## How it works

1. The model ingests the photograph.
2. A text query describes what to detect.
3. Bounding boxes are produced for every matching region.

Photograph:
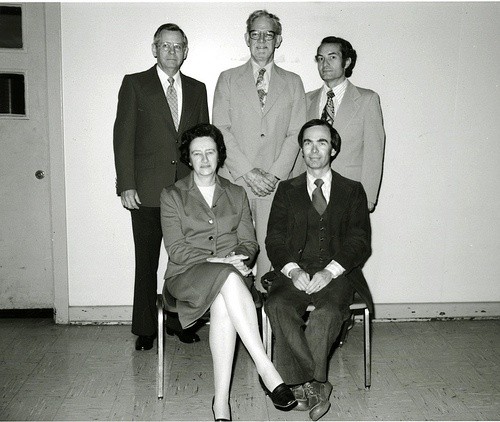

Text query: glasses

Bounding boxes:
[158,43,183,51]
[248,30,278,40]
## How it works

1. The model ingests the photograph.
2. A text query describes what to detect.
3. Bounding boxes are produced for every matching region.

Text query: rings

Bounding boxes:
[318,285,320,288]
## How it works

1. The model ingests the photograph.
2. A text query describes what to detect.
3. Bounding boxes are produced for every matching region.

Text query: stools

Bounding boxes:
[268,293,372,389]
[156,281,269,399]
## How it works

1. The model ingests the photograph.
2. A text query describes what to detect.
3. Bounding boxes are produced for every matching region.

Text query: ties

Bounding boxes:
[256,68,268,108]
[167,77,179,133]
[312,179,327,216]
[321,90,335,127]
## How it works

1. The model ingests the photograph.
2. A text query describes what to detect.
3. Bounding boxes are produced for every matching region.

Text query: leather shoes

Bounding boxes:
[165,318,200,343]
[212,396,232,422]
[259,375,298,412]
[135,334,158,350]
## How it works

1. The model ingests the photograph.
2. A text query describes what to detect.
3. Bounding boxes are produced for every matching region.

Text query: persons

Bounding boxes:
[304,36,385,331]
[263,118,376,421]
[213,10,307,295]
[160,123,299,422]
[113,23,210,350]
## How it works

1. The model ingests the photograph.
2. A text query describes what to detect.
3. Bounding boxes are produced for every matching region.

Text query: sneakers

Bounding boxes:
[302,380,333,421]
[289,384,309,411]
